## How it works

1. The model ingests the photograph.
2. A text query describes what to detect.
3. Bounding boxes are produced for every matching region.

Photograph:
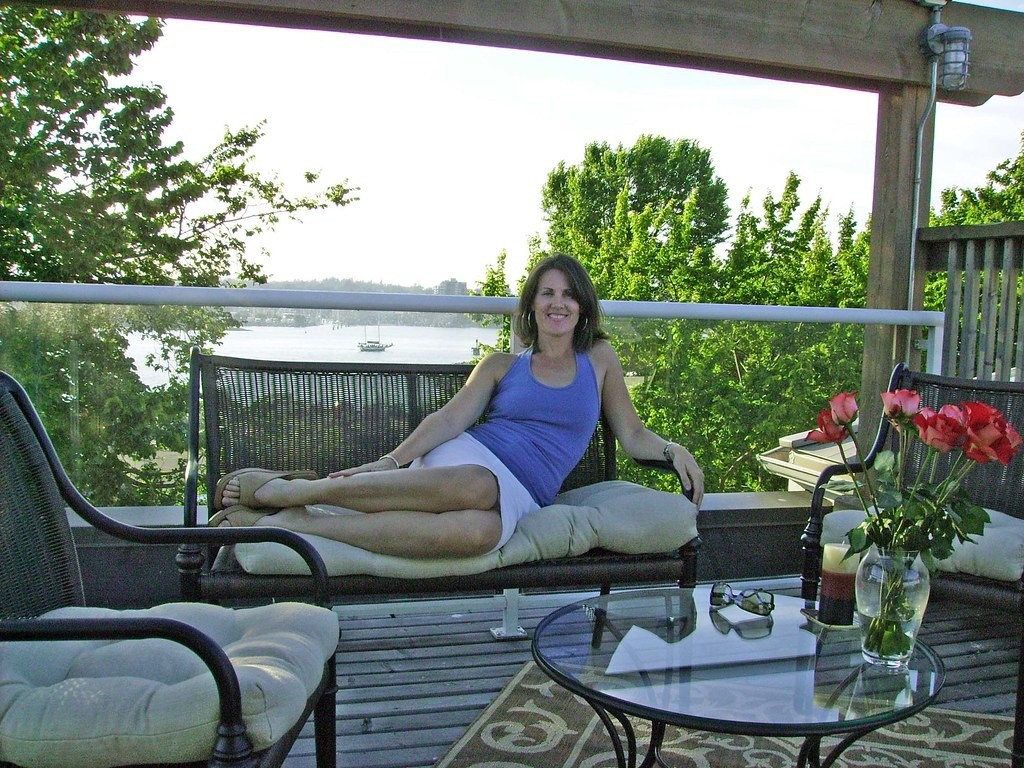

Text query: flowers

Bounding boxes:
[804,388,1024,572]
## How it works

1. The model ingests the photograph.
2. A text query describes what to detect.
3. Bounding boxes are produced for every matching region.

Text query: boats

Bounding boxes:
[360,340,386,352]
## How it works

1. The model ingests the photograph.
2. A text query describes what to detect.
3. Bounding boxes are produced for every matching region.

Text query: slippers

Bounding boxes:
[208,505,287,527]
[213,468,320,510]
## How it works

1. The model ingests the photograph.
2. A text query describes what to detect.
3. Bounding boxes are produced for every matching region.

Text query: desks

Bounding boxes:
[531,588,946,768]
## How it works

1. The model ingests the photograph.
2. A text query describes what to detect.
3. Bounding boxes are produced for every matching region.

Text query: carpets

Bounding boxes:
[433,653,1014,768]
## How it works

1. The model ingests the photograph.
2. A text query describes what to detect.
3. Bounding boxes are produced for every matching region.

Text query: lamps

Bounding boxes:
[925,24,970,89]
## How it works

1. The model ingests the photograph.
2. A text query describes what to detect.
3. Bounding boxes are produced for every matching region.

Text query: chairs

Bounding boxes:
[0,370,341,768]
[796,364,1024,714]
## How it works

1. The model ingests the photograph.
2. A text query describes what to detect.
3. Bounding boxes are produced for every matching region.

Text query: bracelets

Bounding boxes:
[379,456,399,469]
[663,442,676,463]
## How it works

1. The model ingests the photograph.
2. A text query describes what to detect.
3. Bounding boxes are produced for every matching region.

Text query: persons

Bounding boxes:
[209,253,704,558]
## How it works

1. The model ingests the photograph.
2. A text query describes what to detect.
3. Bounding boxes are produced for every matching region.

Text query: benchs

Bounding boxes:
[172,346,703,714]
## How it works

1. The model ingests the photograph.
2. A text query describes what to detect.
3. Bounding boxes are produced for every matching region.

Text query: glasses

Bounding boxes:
[711,611,774,639]
[710,581,775,616]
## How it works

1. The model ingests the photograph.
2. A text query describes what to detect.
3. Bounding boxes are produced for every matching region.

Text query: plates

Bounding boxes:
[800,609,862,631]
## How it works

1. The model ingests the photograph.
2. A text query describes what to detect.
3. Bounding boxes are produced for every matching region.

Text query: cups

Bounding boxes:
[818,542,861,626]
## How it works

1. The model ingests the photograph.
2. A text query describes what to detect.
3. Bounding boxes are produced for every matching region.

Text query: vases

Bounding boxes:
[856,546,931,667]
